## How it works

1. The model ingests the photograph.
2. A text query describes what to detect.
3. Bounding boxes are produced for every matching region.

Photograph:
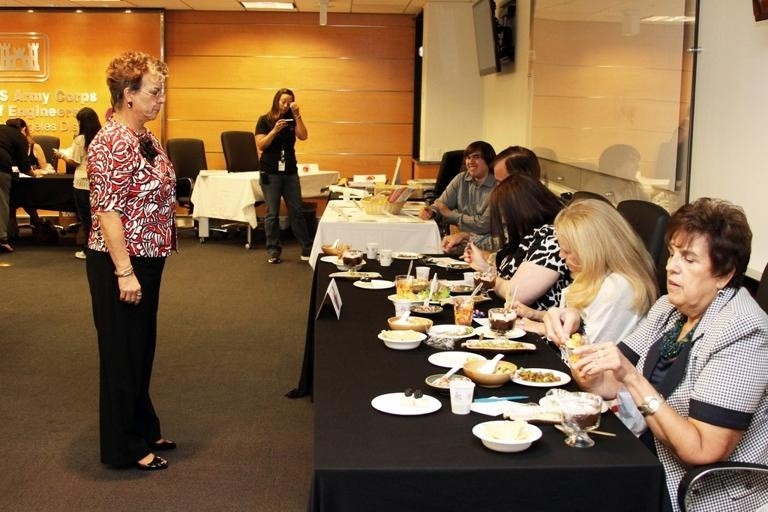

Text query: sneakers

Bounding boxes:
[300,253,310,261]
[75,251,87,259]
[267,255,281,263]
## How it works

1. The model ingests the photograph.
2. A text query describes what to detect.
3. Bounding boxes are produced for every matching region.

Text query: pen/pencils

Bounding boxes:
[510,285,519,309]
[460,242,480,259]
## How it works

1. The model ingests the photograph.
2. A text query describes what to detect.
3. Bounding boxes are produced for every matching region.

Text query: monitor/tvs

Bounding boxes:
[471,1,505,77]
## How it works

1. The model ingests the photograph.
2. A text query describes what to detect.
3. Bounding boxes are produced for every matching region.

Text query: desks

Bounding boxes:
[11,173,78,213]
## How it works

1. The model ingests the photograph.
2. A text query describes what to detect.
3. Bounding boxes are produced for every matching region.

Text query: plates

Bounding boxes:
[473,421,543,451]
[353,280,395,289]
[378,330,427,350]
[466,339,536,351]
[394,252,422,260]
[477,325,527,337]
[370,393,442,416]
[539,396,608,415]
[410,305,443,314]
[328,272,380,278]
[387,294,422,302]
[426,374,471,389]
[429,324,476,338]
[320,256,366,270]
[510,367,571,388]
[448,295,491,304]
[428,351,487,368]
[472,317,490,324]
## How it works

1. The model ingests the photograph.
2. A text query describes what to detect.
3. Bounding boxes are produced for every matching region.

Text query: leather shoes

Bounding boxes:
[153,438,177,449]
[136,453,168,469]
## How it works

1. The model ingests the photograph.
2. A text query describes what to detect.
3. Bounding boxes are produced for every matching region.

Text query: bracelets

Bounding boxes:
[295,115,302,120]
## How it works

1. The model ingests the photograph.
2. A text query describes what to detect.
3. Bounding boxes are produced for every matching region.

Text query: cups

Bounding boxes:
[396,275,414,299]
[417,267,430,281]
[366,243,379,259]
[561,392,602,449]
[453,297,474,326]
[464,272,476,287]
[449,379,476,416]
[380,250,392,267]
[489,307,514,329]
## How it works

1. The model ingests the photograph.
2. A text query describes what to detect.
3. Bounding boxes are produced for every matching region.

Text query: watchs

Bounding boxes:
[113,265,133,277]
[636,392,664,416]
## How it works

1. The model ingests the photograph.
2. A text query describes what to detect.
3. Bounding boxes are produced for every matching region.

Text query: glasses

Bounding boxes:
[137,90,160,99]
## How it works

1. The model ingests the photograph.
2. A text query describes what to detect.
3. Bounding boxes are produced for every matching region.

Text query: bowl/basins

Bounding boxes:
[322,245,339,255]
[387,316,433,333]
[463,359,517,388]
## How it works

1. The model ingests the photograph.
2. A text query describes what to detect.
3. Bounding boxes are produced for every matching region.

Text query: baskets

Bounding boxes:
[361,190,406,214]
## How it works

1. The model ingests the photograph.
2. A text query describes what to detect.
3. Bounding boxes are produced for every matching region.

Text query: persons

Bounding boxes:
[462,172,572,307]
[26,131,47,174]
[256,88,315,263]
[507,197,657,347]
[421,142,496,235]
[575,200,768,512]
[84,50,177,470]
[51,107,101,259]
[0,118,28,253]
[441,146,542,252]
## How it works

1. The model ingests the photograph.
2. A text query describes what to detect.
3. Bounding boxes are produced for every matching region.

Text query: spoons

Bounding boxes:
[478,354,504,373]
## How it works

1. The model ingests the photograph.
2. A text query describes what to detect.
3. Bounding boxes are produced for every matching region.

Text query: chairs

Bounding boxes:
[423,150,465,204]
[18,135,67,236]
[673,264,764,512]
[66,161,83,234]
[219,131,260,249]
[166,138,229,241]
[617,198,671,281]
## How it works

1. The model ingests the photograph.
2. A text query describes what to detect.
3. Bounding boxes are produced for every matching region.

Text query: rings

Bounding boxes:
[137,293,141,296]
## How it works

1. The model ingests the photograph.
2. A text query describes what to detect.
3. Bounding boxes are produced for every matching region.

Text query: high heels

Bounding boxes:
[0,245,15,253]
